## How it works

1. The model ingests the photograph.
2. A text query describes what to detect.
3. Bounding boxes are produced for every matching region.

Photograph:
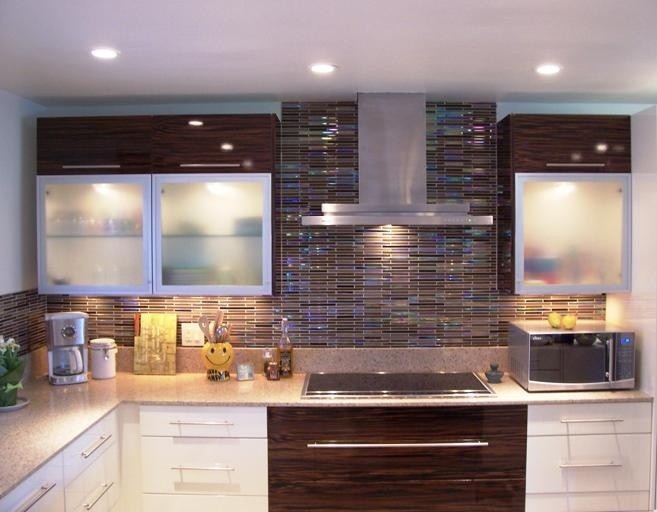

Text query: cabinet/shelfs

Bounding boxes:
[267,404,526,512]
[524,402,653,512]
[496,114,633,294]
[0,408,123,512]
[34,114,151,298]
[151,113,282,299]
[138,404,269,512]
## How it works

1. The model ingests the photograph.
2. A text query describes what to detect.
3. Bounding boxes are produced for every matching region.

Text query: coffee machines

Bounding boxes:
[43,310,90,387]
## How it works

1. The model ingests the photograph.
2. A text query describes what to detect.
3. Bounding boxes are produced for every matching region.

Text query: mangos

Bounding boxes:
[561,316,576,329]
[548,313,561,327]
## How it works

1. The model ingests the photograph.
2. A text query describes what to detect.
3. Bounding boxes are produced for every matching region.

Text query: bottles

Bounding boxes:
[262,316,292,382]
[88,336,117,380]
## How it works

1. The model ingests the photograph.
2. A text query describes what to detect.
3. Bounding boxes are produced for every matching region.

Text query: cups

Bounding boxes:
[48,210,142,237]
[213,265,237,284]
[234,356,254,381]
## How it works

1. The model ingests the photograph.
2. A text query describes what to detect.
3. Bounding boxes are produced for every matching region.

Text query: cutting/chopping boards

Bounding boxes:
[131,309,177,375]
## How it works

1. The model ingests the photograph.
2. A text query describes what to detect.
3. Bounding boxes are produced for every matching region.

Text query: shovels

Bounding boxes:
[197,316,212,343]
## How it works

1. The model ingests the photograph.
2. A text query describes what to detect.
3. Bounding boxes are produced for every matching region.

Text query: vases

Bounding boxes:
[0,382,18,407]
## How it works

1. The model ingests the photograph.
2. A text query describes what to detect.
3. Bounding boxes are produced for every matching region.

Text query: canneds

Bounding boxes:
[267,362,279,381]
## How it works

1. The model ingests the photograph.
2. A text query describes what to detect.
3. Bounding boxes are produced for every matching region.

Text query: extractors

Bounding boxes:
[300,94,493,227]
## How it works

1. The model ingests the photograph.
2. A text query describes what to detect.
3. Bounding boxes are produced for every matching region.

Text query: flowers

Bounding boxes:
[0,334,26,381]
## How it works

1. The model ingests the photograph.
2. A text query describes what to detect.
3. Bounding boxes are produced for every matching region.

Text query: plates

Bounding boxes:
[232,216,262,235]
[161,260,210,287]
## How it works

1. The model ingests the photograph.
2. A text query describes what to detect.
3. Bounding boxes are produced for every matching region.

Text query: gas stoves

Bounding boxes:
[305,371,490,394]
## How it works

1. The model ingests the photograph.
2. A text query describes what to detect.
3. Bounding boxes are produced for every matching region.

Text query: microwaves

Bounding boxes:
[506,319,636,394]
